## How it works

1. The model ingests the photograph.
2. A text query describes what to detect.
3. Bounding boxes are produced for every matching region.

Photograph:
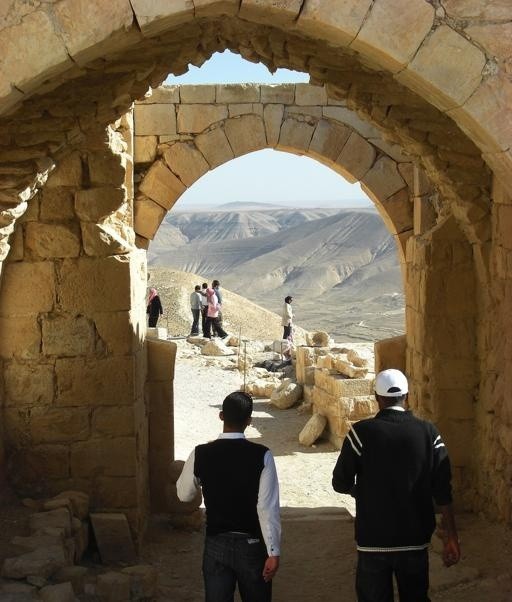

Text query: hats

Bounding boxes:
[373,369,409,398]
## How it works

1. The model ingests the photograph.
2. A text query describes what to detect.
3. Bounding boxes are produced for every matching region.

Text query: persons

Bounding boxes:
[175,391,282,602]
[190,281,228,340]
[147,288,163,328]
[281,296,296,339]
[332,368,460,602]
